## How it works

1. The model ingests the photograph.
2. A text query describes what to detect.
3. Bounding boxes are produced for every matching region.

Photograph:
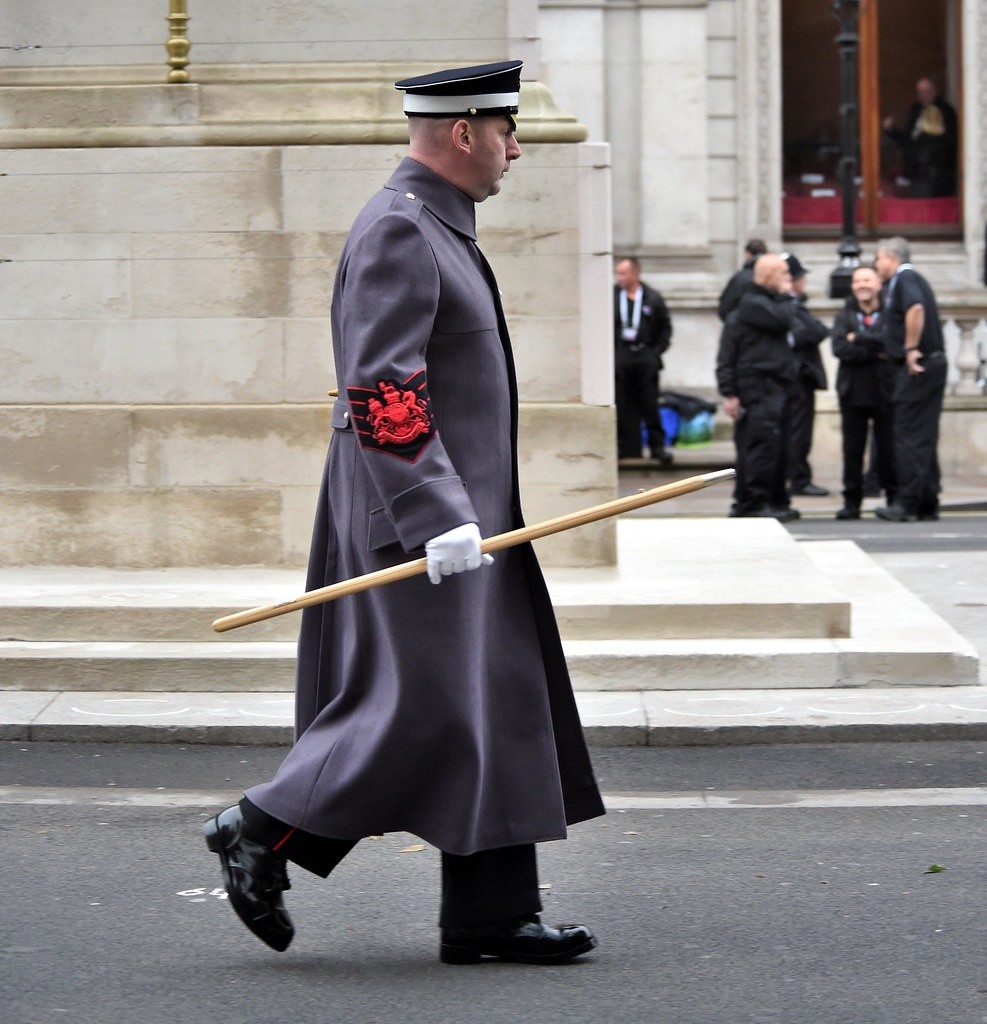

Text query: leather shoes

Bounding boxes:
[204,804,295,952]
[438,925,598,965]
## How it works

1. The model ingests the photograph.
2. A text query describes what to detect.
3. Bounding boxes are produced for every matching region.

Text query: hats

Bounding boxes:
[394,59,524,131]
[785,254,808,279]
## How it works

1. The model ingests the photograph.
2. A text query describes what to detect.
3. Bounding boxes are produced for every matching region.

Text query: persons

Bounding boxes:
[201,60,613,964]
[881,76,956,199]
[713,236,950,524]
[613,257,675,469]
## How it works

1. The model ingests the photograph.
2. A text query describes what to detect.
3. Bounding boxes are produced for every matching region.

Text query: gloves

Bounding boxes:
[425,523,494,585]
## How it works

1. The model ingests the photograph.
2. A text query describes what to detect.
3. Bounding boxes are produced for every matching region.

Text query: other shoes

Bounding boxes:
[876,503,911,522]
[729,507,802,523]
[650,449,673,463]
[836,507,861,520]
[841,480,881,498]
[789,483,829,495]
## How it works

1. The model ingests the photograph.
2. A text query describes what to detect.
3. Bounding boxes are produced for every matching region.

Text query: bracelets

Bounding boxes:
[903,346,918,353]
